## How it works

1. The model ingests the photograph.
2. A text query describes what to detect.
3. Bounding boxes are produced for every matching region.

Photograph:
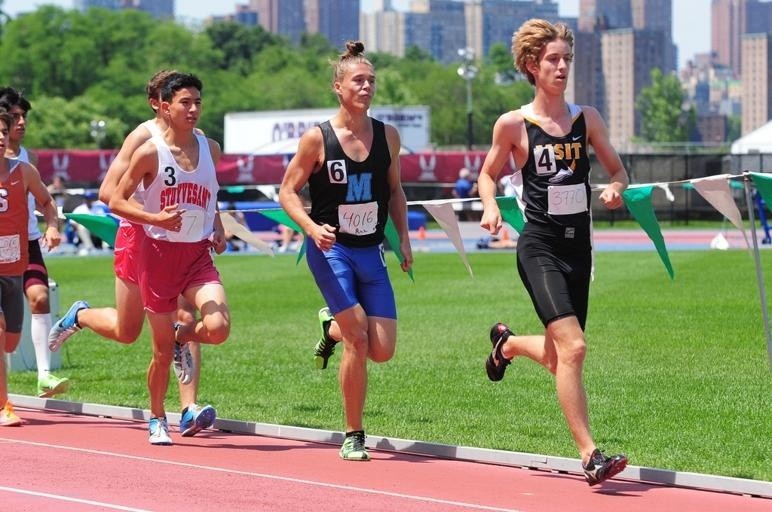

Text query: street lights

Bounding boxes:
[91,117,109,149]
[458,46,480,147]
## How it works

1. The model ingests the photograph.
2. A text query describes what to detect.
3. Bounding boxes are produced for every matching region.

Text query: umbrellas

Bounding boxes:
[724,116,772,159]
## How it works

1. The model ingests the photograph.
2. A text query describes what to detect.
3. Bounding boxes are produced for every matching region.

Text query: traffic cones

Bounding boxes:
[418,225,427,241]
[503,230,510,239]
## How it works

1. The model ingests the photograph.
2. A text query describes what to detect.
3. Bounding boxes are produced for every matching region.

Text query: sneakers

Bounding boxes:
[149,416,173,444]
[339,430,370,461]
[37,374,69,398]
[174,323,194,384]
[581,448,627,487]
[0,405,23,426]
[180,403,216,437]
[486,322,517,382]
[48,300,90,352]
[313,307,340,370]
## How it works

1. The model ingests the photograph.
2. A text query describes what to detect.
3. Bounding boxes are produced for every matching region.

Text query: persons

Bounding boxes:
[108,71,234,446]
[453,170,483,220]
[476,237,517,249]
[278,39,416,462]
[208,188,312,250]
[473,15,629,489]
[498,174,517,197]
[408,206,427,240]
[1,109,64,429]
[48,176,115,249]
[1,88,72,401]
[48,65,217,437]
